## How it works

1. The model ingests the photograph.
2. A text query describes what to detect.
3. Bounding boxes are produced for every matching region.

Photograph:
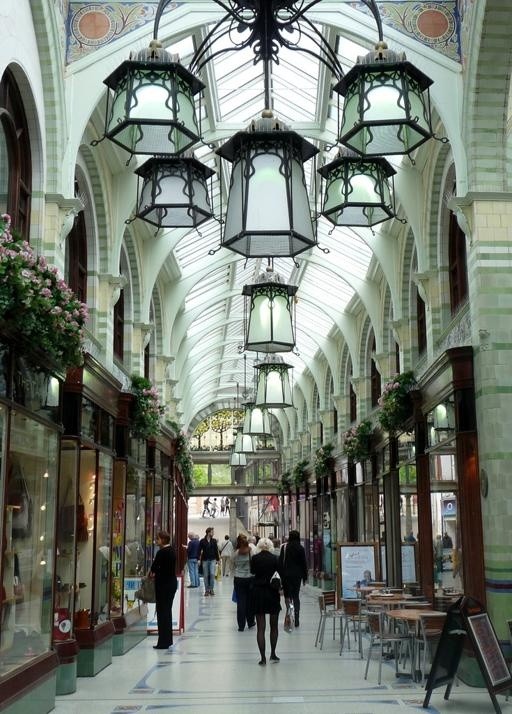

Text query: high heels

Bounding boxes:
[258,659,267,667]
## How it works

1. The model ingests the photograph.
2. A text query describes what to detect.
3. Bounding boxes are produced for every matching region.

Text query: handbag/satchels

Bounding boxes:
[284,604,294,633]
[136,575,156,604]
[270,570,283,590]
[218,550,223,555]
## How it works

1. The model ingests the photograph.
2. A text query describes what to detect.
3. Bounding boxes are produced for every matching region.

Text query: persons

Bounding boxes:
[228,534,256,632]
[246,537,282,665]
[435,534,442,546]
[186,495,288,595]
[354,570,375,586]
[278,529,308,627]
[149,529,178,649]
[442,531,452,548]
[380,531,385,543]
[404,529,416,541]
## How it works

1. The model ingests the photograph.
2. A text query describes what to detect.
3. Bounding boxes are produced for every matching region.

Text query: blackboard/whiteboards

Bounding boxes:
[459,595,512,695]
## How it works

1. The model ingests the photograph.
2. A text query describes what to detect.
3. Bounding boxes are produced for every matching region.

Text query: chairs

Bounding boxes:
[314,577,451,688]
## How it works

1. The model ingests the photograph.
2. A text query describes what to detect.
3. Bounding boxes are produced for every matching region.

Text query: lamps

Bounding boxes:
[433,403,449,432]
[39,375,60,410]
[225,270,302,469]
[91,0,449,271]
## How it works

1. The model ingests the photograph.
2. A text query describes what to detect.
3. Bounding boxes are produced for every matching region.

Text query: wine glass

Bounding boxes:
[380,586,391,593]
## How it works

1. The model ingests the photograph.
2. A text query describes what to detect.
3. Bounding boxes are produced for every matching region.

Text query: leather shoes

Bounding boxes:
[211,589,216,596]
[186,586,196,588]
[204,590,210,596]
[270,655,280,664]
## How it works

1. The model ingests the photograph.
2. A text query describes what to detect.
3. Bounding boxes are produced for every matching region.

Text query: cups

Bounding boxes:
[64,583,70,592]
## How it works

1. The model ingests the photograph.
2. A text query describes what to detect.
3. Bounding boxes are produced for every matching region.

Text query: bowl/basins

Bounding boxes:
[371,589,379,593]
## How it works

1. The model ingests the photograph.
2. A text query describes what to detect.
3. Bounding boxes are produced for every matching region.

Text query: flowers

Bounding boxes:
[125,373,166,443]
[0,213,91,375]
[173,427,196,495]
[275,371,424,493]
